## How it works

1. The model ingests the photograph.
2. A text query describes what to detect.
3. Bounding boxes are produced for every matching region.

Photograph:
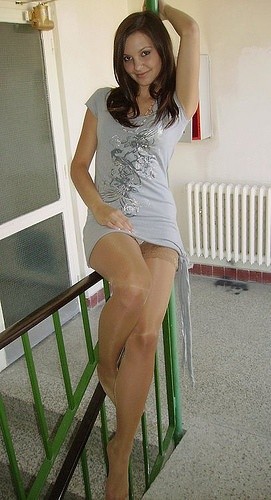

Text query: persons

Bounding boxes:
[69,0,198,500]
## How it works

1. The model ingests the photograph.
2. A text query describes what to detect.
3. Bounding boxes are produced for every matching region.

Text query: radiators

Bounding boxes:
[185,181,271,267]
